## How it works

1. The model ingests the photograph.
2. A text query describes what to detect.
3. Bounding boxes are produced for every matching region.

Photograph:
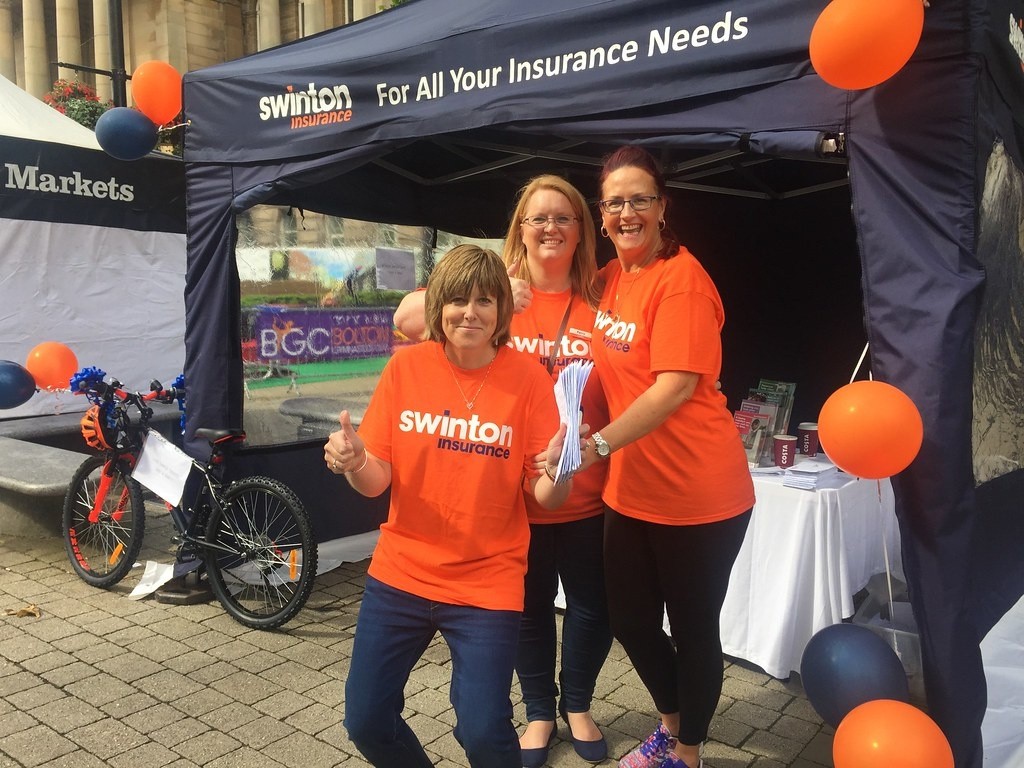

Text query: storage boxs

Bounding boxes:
[858,592,921,673]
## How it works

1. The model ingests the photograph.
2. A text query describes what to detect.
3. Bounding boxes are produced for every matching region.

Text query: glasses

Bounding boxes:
[598,195,660,213]
[522,213,580,229]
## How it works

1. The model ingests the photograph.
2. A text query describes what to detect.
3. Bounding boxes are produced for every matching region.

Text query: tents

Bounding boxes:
[185,0,1024,768]
[0,68,188,409]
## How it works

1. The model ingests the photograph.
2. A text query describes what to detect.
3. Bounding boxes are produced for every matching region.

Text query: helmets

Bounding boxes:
[82,405,119,451]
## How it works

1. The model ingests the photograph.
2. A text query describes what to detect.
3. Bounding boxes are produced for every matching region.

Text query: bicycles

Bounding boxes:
[63,378,318,630]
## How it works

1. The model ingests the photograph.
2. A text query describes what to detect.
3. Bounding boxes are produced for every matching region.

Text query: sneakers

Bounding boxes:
[658,752,702,768]
[618,721,703,768]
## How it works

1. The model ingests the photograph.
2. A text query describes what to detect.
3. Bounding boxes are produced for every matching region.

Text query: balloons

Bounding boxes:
[804,625,916,714]
[1,360,40,410]
[131,59,182,129]
[816,379,926,479]
[27,341,79,392]
[802,0,924,95]
[95,107,161,161]
[821,703,954,766]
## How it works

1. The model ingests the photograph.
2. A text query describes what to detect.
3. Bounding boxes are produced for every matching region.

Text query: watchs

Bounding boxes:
[591,432,610,456]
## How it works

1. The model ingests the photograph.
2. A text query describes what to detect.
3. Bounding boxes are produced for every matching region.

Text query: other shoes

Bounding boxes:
[519,719,558,768]
[558,704,608,763]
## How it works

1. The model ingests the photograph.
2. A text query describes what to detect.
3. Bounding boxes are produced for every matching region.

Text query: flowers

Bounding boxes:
[128,105,186,157]
[45,63,115,130]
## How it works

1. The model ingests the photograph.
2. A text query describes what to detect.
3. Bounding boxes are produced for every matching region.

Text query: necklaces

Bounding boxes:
[443,349,497,410]
[614,258,646,320]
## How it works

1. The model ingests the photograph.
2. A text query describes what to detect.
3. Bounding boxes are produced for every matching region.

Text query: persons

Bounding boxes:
[324,243,593,768]
[587,143,756,768]
[401,174,616,768]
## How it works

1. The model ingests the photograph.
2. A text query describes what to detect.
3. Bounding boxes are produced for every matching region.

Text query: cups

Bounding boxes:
[798,422,819,455]
[773,434,797,468]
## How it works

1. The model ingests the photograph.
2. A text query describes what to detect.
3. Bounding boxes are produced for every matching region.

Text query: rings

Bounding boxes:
[584,440,591,450]
[331,460,338,471]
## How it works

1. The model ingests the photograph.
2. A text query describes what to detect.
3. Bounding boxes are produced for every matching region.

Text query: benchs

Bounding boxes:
[0,400,188,539]
[280,398,368,442]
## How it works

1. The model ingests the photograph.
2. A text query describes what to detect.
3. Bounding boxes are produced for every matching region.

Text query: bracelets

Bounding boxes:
[351,449,368,474]
[544,467,575,486]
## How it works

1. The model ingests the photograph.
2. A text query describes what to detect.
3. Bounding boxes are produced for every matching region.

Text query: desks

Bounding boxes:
[554,447,907,682]
